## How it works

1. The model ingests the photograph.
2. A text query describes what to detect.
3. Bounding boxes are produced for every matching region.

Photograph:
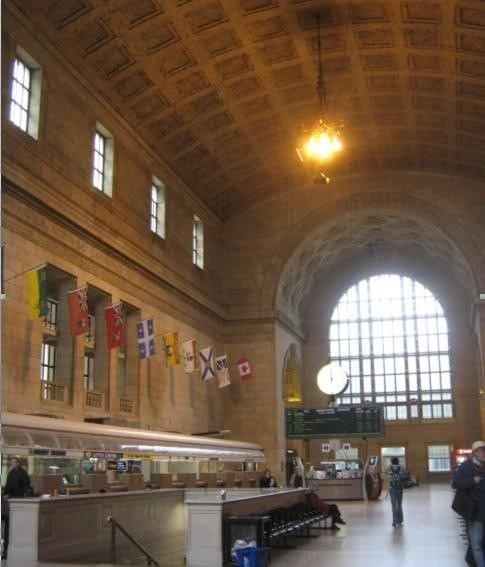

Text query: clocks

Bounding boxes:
[318,364,348,395]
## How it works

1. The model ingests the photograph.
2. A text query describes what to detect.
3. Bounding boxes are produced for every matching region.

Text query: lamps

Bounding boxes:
[294,22,345,183]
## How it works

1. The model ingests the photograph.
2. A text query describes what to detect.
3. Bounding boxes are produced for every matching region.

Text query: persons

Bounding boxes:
[312,487,346,530]
[452,440,485,567]
[260,470,277,487]
[1,458,34,561]
[385,457,404,527]
[287,466,302,489]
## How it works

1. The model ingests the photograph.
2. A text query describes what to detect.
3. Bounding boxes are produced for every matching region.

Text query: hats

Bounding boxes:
[472,441,485,451]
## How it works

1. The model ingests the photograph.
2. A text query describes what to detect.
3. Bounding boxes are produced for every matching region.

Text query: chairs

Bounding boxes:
[236,499,331,551]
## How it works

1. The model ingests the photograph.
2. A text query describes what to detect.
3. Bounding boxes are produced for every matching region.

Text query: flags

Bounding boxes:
[163,331,179,369]
[198,347,215,382]
[183,339,200,374]
[136,318,155,359]
[214,355,231,390]
[105,303,128,352]
[25,264,49,321]
[67,288,89,337]
[239,356,256,384]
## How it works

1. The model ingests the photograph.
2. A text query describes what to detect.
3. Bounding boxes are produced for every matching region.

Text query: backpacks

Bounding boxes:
[390,467,400,489]
